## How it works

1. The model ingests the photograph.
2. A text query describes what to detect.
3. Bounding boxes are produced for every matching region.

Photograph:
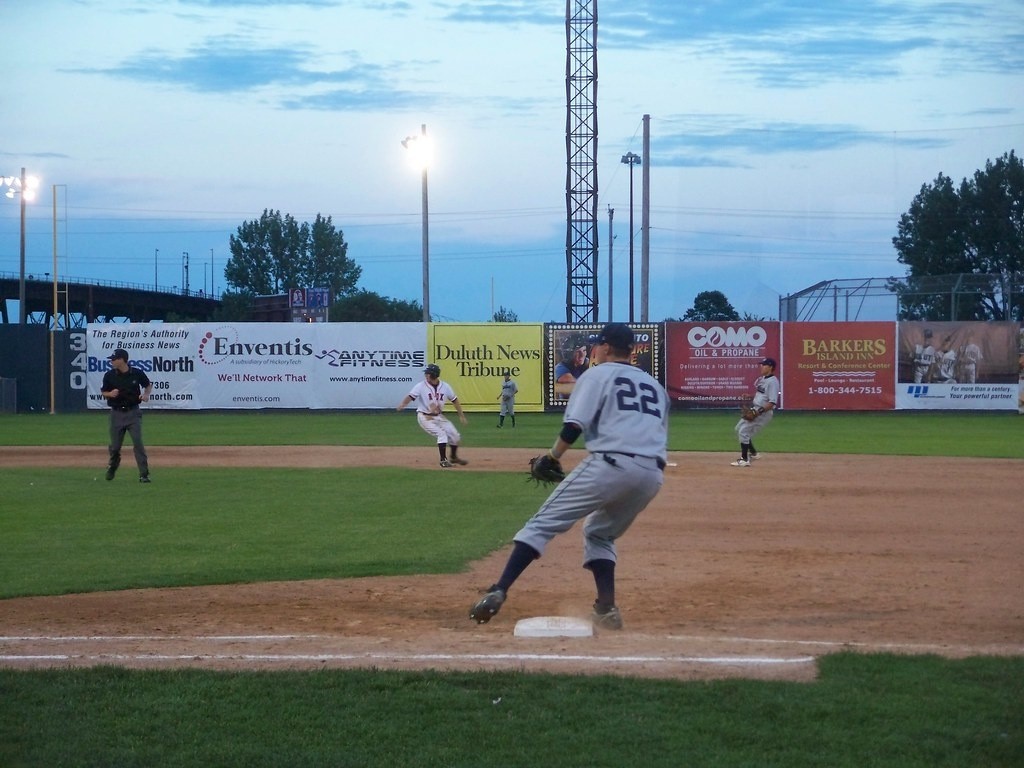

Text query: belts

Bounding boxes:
[423,413,441,416]
[622,453,665,470]
[113,404,139,410]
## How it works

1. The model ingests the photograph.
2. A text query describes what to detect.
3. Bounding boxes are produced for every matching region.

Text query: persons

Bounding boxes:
[397,364,468,468]
[470,322,670,631]
[496,372,519,428]
[100,349,152,484]
[910,330,981,384]
[555,333,590,398]
[731,358,780,467]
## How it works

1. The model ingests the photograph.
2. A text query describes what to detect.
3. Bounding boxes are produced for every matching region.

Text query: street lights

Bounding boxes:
[155,248,159,292]
[401,124,435,322]
[210,248,214,300]
[619,151,643,324]
[204,262,207,298]
[0,166,40,321]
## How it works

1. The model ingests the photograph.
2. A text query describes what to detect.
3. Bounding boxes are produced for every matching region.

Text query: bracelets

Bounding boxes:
[550,448,560,460]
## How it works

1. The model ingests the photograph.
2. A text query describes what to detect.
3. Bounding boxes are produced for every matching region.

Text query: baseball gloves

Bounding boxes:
[740,408,759,421]
[529,454,565,483]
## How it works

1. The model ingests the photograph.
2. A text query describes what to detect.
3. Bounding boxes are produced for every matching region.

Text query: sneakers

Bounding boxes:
[731,457,751,467]
[591,599,623,631]
[469,583,507,625]
[749,453,760,460]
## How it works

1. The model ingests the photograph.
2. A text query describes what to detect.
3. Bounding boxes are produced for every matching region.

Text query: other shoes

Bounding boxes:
[449,457,468,465]
[140,476,151,483]
[497,424,504,429]
[512,423,516,427]
[440,461,452,467]
[105,465,119,480]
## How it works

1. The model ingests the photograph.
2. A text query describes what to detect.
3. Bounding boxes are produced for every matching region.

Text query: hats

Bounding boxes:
[922,329,933,339]
[505,372,511,376]
[759,358,776,368]
[589,323,636,353]
[423,363,441,377]
[107,349,128,359]
